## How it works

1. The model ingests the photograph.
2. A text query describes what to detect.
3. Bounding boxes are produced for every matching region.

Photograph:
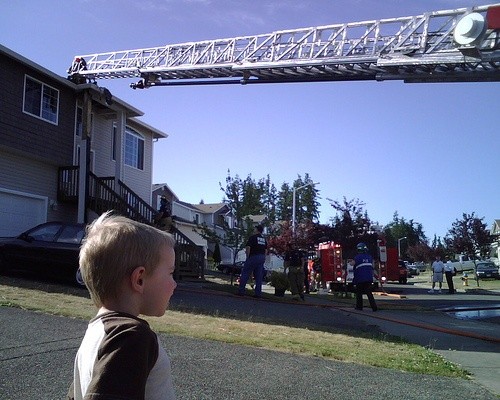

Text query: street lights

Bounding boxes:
[398,236,407,257]
[291,181,320,244]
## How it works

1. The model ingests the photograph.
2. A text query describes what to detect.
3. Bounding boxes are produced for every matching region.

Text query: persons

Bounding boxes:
[442,256,456,294]
[428,254,445,294]
[232,224,268,299]
[67,210,178,400]
[351,243,382,313]
[283,242,322,302]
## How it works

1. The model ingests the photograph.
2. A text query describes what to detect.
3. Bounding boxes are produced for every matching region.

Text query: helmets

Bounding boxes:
[357,243,369,251]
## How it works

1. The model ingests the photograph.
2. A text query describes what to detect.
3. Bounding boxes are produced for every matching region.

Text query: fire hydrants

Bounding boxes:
[460,274,469,286]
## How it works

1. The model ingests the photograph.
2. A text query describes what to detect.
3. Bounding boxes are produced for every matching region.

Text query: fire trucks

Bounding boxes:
[318,234,408,292]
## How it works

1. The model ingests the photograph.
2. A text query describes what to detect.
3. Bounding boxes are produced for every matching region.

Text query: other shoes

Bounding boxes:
[252,295,263,298]
[372,305,378,312]
[355,307,363,311]
[428,290,434,293]
[234,292,245,296]
[439,290,442,293]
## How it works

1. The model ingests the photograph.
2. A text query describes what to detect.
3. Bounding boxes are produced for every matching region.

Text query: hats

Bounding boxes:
[255,225,263,233]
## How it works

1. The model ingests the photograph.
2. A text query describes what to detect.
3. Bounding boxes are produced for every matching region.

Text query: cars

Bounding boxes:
[406,254,481,276]
[475,263,499,280]
[217,259,245,275]
[0,219,90,289]
